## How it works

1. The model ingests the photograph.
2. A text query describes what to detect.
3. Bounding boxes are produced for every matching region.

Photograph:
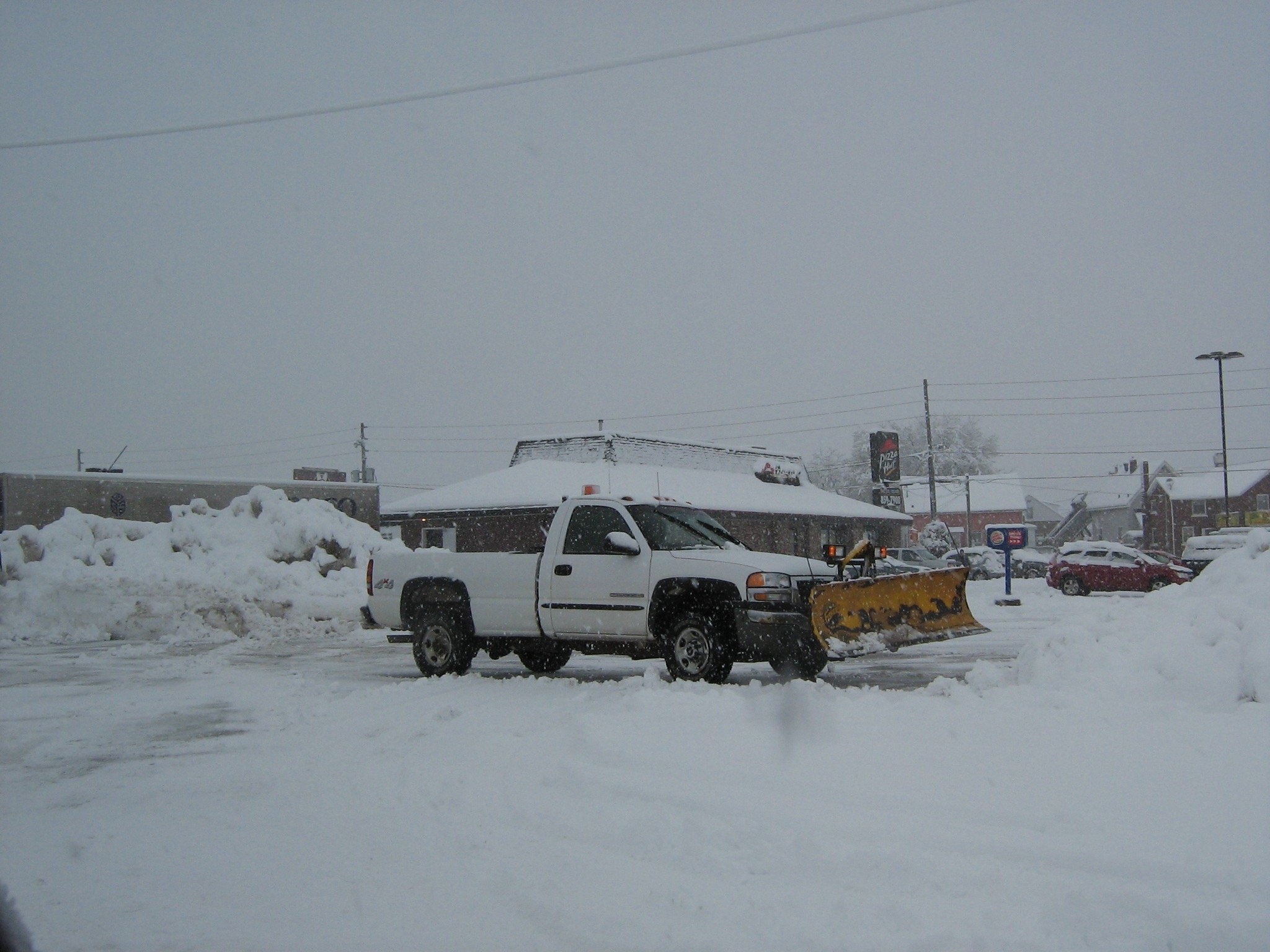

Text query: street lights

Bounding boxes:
[1194,351,1244,527]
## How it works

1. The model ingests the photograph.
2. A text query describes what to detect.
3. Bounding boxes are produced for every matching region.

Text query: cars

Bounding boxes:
[1139,534,1246,578]
[854,546,1050,581]
[1045,544,1194,596]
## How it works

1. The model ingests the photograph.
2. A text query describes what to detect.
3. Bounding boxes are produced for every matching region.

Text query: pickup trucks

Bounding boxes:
[358,494,851,686]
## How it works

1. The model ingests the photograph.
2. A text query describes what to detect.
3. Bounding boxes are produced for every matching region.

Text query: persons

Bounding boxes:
[956,549,971,580]
